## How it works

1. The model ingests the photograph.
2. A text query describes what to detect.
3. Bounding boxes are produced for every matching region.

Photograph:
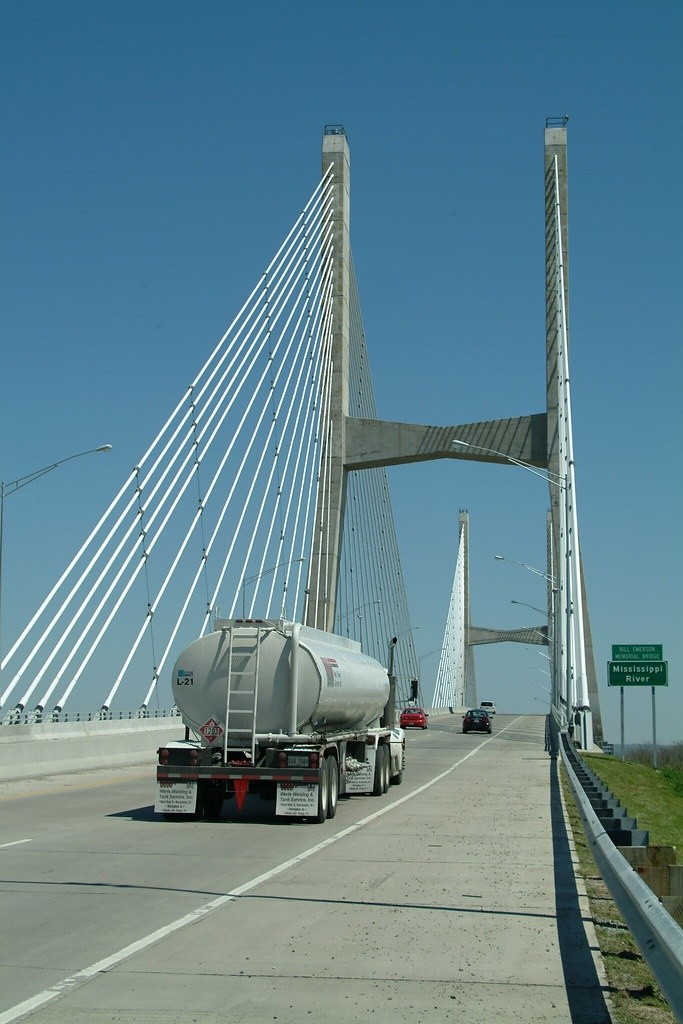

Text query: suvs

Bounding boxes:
[480,701,496,714]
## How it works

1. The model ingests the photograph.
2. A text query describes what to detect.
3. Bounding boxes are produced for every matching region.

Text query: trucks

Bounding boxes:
[154,613,406,823]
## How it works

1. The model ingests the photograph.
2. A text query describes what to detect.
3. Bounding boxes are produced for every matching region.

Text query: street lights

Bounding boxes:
[0,443,113,567]
[243,556,306,618]
[452,440,576,725]
[511,601,558,709]
[418,648,446,707]
[337,600,381,636]
[495,556,562,709]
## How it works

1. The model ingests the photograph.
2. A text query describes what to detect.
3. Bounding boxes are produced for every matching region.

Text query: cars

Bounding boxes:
[400,708,429,730]
[462,709,492,734]
